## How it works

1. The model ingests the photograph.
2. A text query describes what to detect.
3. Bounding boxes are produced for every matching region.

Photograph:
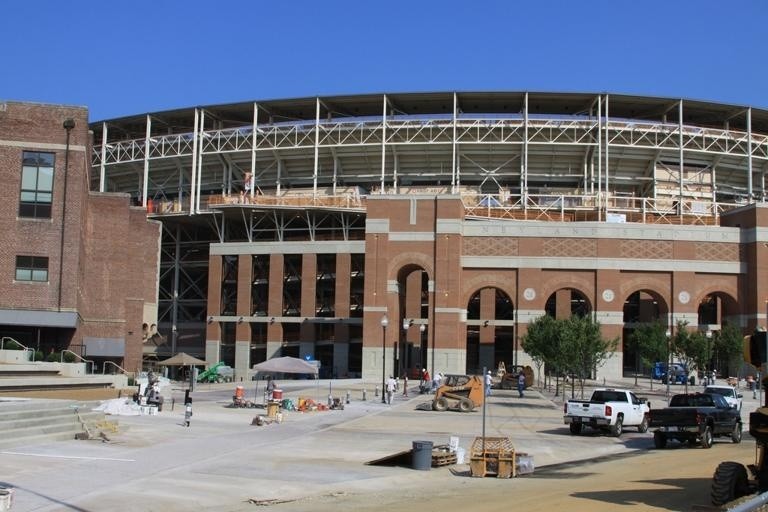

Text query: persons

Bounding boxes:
[433,372,443,391]
[141,322,149,340]
[385,373,397,406]
[515,370,526,398]
[422,367,432,393]
[485,370,493,398]
[711,368,716,386]
[267,376,276,401]
[147,323,156,339]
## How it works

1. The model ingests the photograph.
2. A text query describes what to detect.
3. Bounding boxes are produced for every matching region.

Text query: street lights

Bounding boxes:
[665,325,672,396]
[381,312,387,403]
[705,328,712,385]
[419,321,424,390]
[403,317,411,396]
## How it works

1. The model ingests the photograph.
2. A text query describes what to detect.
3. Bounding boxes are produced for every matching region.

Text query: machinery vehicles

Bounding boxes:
[723,375,738,387]
[416,373,485,411]
[490,365,535,391]
[651,361,696,385]
[711,329,768,511]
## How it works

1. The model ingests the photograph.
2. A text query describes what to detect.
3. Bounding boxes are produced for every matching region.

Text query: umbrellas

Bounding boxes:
[154,352,209,391]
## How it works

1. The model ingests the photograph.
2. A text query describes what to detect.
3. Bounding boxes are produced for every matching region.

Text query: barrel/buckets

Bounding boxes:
[274,390,283,401]
[268,402,280,418]
[236,386,244,399]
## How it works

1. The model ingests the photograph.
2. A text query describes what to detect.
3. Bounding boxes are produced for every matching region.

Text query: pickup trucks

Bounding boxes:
[562,386,652,437]
[647,392,745,449]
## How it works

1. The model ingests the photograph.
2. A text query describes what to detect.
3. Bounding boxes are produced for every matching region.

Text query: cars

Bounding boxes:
[700,385,744,414]
